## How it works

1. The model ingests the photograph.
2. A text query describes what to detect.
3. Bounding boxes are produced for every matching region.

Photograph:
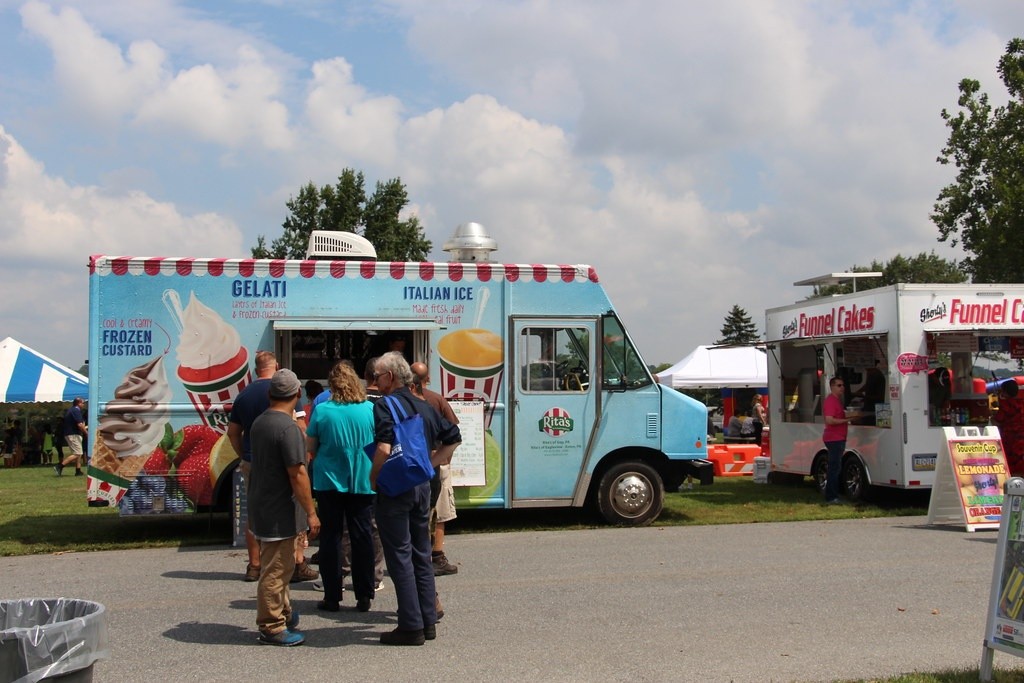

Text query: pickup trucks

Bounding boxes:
[712,422,724,433]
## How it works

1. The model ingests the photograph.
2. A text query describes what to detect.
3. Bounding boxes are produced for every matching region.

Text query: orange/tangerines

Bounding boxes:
[210,432,239,488]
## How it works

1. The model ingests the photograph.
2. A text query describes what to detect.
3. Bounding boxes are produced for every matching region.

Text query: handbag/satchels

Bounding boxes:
[363,397,435,496]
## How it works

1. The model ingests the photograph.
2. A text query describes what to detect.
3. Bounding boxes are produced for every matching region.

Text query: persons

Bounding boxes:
[729,394,767,446]
[856,359,885,412]
[989,379,1024,478]
[53,398,88,477]
[822,377,855,503]
[228,338,459,613]
[249,369,321,647]
[370,351,463,647]
[1,410,88,477]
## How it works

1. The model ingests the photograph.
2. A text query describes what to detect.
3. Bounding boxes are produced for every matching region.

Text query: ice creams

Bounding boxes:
[177,291,248,384]
[89,356,172,485]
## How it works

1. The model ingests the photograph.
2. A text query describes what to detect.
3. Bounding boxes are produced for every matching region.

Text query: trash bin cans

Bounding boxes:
[0,598,105,683]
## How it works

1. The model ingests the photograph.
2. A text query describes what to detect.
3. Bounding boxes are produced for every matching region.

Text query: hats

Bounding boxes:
[366,358,380,375]
[270,368,301,396]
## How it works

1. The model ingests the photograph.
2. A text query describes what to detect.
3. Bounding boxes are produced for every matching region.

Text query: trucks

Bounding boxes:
[88,222,711,526]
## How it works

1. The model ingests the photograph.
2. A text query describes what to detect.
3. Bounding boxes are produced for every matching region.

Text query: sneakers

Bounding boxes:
[246,563,261,581]
[54,465,61,477]
[397,592,444,621]
[257,628,305,647]
[423,624,436,640]
[75,471,83,476]
[286,601,299,629]
[289,556,319,583]
[432,554,457,576]
[380,626,425,646]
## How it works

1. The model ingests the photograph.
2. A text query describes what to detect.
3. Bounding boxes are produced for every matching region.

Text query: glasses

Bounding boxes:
[82,403,85,404]
[374,371,389,381]
[833,384,845,387]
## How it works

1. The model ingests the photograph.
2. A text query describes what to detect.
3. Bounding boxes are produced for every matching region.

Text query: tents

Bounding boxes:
[0,337,90,441]
[640,342,767,404]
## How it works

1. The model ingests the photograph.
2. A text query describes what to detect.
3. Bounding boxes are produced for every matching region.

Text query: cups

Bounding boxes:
[175,346,252,439]
[436,343,504,431]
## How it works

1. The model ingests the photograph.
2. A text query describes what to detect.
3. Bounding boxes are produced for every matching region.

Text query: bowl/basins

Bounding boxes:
[847,407,862,418]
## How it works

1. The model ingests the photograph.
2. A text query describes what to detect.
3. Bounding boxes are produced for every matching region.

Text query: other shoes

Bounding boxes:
[826,496,847,506]
[374,581,384,592]
[357,599,371,611]
[317,600,340,611]
[312,580,345,592]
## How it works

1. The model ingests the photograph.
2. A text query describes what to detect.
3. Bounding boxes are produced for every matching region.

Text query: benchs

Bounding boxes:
[724,436,756,444]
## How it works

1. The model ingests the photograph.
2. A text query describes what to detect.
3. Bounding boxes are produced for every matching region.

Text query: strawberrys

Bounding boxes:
[139,425,226,506]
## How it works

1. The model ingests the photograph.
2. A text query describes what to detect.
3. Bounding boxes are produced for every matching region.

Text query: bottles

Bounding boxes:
[930,406,970,426]
